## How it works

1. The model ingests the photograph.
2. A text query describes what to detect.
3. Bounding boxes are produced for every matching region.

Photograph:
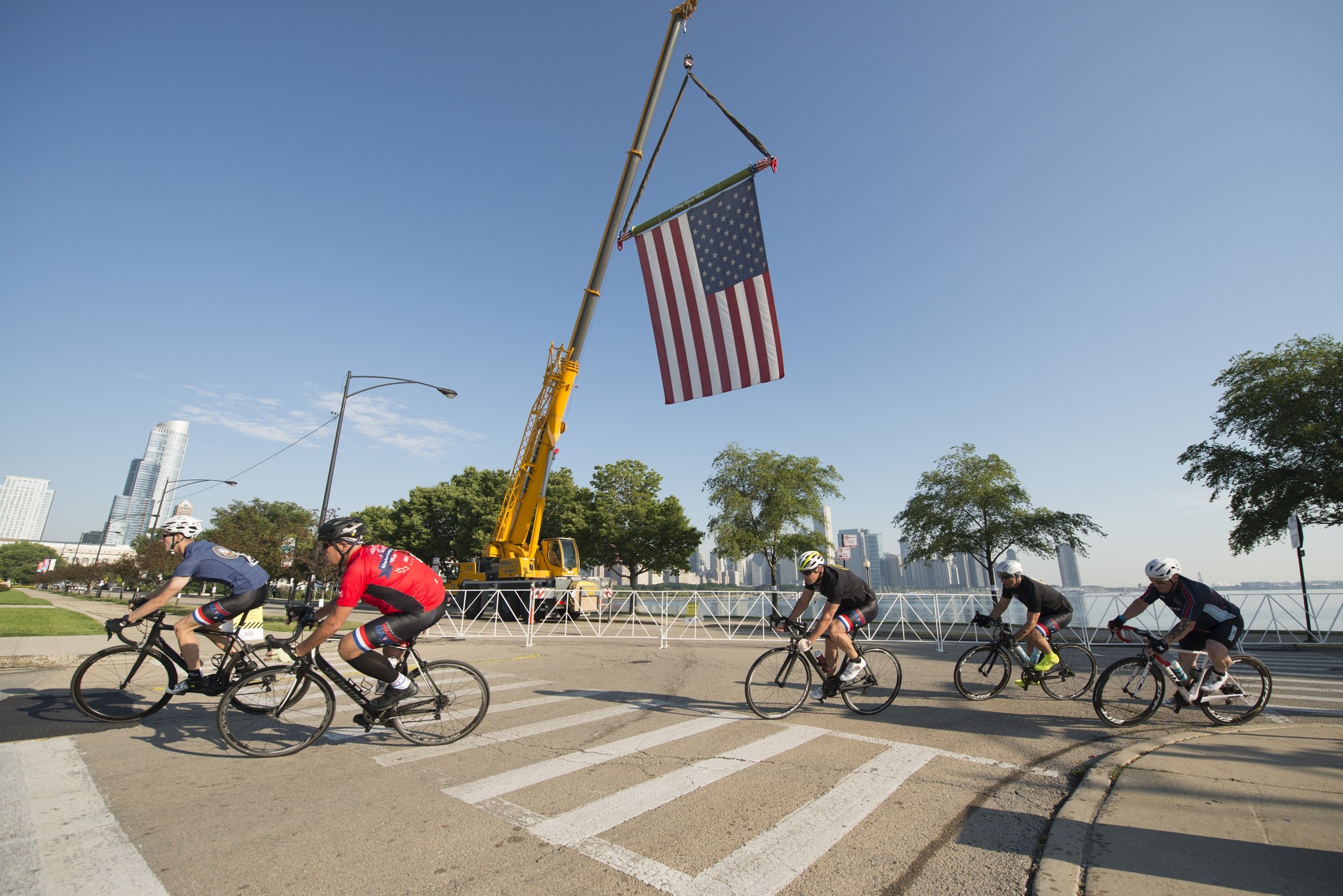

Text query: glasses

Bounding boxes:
[322,540,346,551]
[1148,574,1175,584]
[800,565,822,575]
[162,534,178,538]
[999,574,1013,579]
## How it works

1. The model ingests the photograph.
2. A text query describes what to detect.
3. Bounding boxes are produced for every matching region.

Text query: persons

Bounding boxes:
[1109,558,1245,712]
[105,516,271,693]
[975,561,1073,690]
[266,516,445,727]
[773,550,878,701]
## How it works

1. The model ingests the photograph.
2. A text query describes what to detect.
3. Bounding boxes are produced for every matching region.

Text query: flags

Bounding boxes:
[633,167,787,408]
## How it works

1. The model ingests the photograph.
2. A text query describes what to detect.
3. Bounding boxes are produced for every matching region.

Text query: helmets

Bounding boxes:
[798,551,824,570]
[996,560,1023,575]
[317,515,364,543]
[160,515,203,540]
[1145,557,1181,579]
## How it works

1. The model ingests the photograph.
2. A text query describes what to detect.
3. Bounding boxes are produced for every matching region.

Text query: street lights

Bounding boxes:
[863,557,871,588]
[39,512,161,599]
[130,477,238,604]
[304,370,459,605]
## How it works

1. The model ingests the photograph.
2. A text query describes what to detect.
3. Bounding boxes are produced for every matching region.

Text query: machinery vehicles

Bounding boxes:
[443,1,700,624]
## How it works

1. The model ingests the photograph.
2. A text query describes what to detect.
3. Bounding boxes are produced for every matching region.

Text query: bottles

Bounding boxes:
[376,657,398,693]
[179,652,204,667]
[814,650,832,673]
[1169,660,1187,681]
[1013,644,1031,664]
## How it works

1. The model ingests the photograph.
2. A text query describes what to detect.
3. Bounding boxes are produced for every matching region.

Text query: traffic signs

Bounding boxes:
[841,534,858,548]
[837,547,852,560]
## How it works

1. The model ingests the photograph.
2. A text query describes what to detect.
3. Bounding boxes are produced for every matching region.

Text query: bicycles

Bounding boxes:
[954,612,1096,701]
[217,597,491,758]
[1092,622,1273,728]
[70,595,317,722]
[745,615,902,720]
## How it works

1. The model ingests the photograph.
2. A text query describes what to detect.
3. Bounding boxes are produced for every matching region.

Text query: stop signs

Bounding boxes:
[1288,514,1300,549]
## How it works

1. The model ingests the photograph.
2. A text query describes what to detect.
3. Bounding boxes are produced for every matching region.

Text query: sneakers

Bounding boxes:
[1161,697,1177,709]
[166,670,204,693]
[1015,680,1040,688]
[368,680,417,711]
[839,656,866,681]
[1200,671,1228,691]
[231,661,260,683]
[1035,652,1059,670]
[809,684,825,699]
[352,714,394,729]
